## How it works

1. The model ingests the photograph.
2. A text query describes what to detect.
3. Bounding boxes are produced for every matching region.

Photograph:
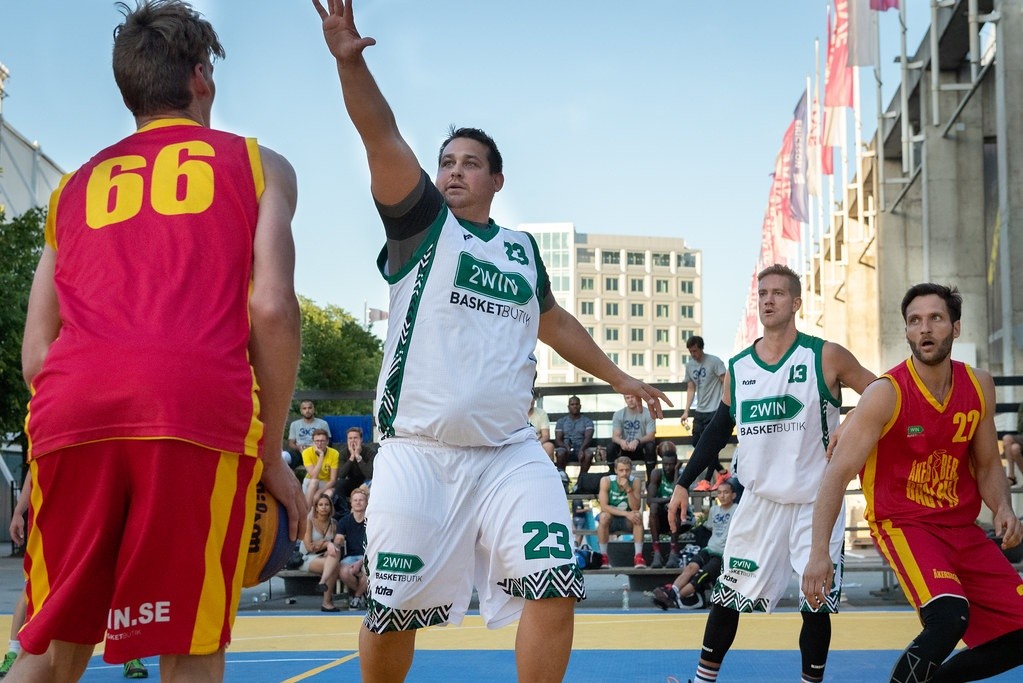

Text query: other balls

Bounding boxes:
[658,440,677,458]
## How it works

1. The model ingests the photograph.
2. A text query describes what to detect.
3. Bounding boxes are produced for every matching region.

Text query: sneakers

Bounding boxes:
[0,652,18,677]
[650,555,665,568]
[709,469,732,491]
[667,552,680,568]
[634,553,647,568]
[123,659,148,677]
[600,553,611,568]
[693,480,711,491]
[348,596,362,609]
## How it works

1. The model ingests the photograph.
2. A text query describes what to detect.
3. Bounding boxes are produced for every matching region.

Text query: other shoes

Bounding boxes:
[315,584,328,592]
[648,586,676,610]
[321,606,340,612]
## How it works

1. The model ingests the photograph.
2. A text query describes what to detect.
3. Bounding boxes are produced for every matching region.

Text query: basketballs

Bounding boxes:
[243,483,298,589]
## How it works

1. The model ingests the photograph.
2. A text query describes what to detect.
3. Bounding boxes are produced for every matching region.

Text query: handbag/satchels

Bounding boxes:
[288,552,304,569]
[578,473,608,494]
[574,541,602,569]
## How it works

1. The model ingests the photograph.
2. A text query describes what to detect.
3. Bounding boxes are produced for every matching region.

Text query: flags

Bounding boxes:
[740,0,897,347]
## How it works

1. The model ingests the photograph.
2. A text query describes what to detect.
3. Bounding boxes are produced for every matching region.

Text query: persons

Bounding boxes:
[312,0,673,683]
[282,399,376,614]
[802,284,1023,683]
[0,468,149,678]
[526,392,740,609]
[668,266,878,683]
[681,335,732,491]
[1003,403,1023,487]
[4,0,308,683]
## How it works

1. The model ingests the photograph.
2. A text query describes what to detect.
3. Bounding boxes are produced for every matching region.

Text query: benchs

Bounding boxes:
[265,376,1023,600]
